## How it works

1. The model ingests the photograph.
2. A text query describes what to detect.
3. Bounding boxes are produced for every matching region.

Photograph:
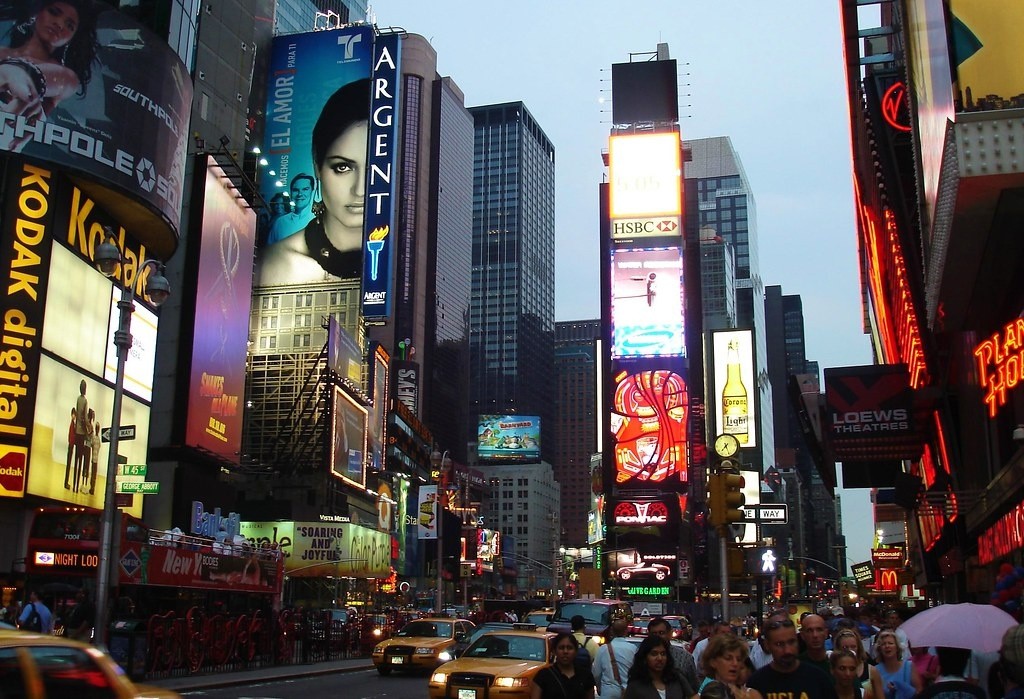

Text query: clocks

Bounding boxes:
[714,433,740,460]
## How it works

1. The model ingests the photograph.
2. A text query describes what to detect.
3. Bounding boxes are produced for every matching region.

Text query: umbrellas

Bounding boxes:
[893,602,1019,684]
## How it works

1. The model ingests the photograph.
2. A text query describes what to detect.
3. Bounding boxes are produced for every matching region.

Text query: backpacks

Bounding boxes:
[20,602,42,633]
[575,637,591,669]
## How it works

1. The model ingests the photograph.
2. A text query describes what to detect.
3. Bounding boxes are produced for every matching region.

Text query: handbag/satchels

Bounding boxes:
[621,688,626,699]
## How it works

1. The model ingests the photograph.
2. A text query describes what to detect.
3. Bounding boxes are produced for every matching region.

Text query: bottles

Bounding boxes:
[241,560,260,584]
[722,340,749,443]
[140,545,151,583]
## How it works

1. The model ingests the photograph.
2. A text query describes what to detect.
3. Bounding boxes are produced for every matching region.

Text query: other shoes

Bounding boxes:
[64,484,94,495]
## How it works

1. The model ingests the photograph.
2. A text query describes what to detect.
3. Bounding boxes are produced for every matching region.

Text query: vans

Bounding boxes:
[546,598,634,645]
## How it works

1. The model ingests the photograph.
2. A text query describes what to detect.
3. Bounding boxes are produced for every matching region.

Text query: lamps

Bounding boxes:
[678,63,691,118]
[599,68,613,124]
[891,473,985,518]
[207,134,295,206]
[196,138,205,153]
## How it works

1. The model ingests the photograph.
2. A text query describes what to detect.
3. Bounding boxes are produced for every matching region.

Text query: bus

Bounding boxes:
[12,505,283,618]
[481,599,553,622]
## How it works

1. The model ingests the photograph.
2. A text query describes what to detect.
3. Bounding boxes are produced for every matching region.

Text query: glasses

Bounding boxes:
[651,630,670,635]
[763,618,793,633]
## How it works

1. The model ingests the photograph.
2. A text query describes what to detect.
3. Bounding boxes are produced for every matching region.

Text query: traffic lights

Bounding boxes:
[497,556,503,569]
[719,472,746,525]
[746,546,779,576]
[706,473,727,525]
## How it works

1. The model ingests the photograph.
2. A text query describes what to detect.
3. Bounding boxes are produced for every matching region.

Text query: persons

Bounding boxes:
[253,79,372,287]
[505,610,1024,699]
[0,0,98,154]
[0,587,97,644]
[176,525,278,562]
[64,379,101,495]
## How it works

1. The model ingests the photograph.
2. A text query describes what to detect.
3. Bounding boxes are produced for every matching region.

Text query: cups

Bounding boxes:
[636,437,661,480]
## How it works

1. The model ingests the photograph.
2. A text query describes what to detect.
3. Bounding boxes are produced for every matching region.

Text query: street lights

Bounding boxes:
[429,441,452,612]
[329,532,342,606]
[432,556,454,606]
[91,233,171,647]
[560,545,566,600]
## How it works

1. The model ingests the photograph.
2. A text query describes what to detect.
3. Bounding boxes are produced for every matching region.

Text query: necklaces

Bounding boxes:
[304,215,363,280]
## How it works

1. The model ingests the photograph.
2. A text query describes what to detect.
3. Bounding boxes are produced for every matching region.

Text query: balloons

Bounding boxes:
[991,563,1024,612]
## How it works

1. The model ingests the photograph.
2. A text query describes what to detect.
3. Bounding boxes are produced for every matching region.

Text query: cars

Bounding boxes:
[454,622,515,659]
[291,607,470,648]
[428,622,559,699]
[628,609,693,642]
[372,618,476,674]
[617,561,670,580]
[520,607,556,633]
[0,627,181,699]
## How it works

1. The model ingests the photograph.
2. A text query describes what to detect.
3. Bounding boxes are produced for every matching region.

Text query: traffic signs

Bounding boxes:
[115,453,160,494]
[99,425,135,443]
[732,503,788,525]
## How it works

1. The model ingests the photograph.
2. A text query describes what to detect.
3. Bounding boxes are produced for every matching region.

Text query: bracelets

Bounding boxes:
[0,57,48,97]
